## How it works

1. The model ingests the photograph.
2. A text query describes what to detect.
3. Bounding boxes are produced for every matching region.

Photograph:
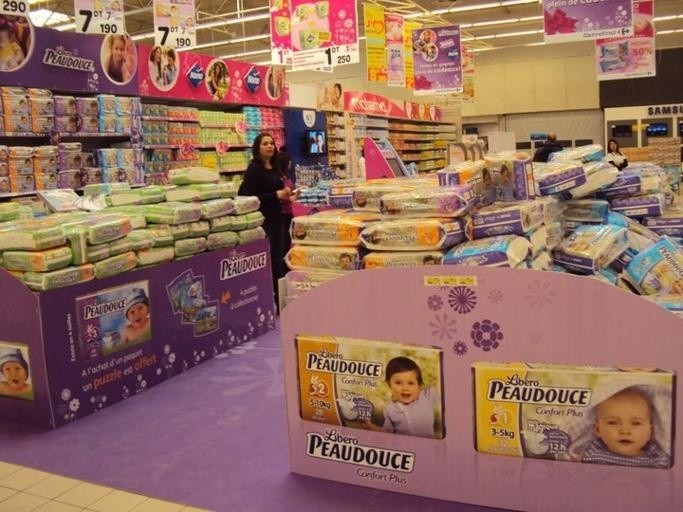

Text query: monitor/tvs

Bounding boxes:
[645,123,668,137]
[611,124,633,138]
[304,128,328,159]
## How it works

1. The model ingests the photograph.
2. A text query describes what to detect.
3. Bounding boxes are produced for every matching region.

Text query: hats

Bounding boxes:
[124,287,150,319]
[0,348,28,371]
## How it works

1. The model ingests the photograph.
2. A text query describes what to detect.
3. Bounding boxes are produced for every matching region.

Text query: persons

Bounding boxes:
[279,146,296,184]
[416,31,435,58]
[482,167,496,204]
[0,23,25,69]
[102,35,130,82]
[606,139,628,170]
[120,287,151,342]
[354,192,366,206]
[238,134,291,316]
[331,83,341,106]
[0,99,101,192]
[277,151,308,252]
[0,346,32,396]
[163,47,177,86]
[310,133,323,152]
[295,225,307,239]
[635,19,651,33]
[532,133,561,162]
[207,64,222,96]
[150,46,163,87]
[669,280,683,296]
[581,389,672,469]
[497,164,514,201]
[340,253,353,270]
[268,73,282,97]
[423,255,436,264]
[360,357,442,437]
